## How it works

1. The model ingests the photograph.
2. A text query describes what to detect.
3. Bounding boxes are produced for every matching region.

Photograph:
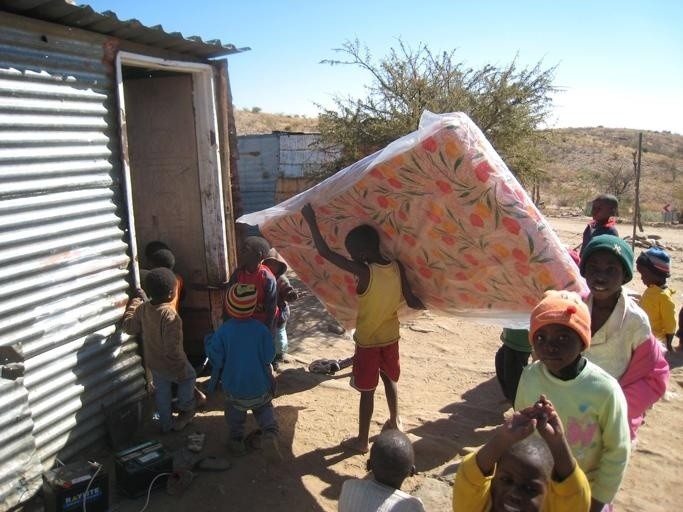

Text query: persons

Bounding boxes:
[206,234,296,465]
[300,201,429,455]
[574,194,622,248]
[632,245,676,350]
[452,391,593,510]
[510,287,632,512]
[336,428,427,512]
[576,231,670,446]
[123,239,197,437]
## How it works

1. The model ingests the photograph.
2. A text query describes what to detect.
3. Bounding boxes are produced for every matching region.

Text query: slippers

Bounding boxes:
[343,436,369,453]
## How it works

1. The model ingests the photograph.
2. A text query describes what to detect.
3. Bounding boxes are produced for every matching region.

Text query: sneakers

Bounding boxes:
[167,432,246,493]
[247,429,283,463]
[310,359,340,374]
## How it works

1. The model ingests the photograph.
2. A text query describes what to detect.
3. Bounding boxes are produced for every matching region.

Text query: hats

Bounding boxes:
[636,246,671,278]
[579,233,633,285]
[224,282,258,318]
[529,289,592,352]
